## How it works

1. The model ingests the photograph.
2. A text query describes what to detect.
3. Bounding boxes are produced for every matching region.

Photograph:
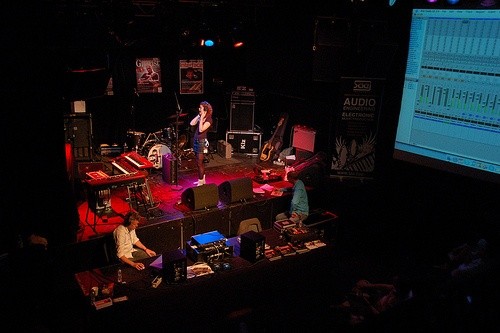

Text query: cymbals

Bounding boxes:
[170,114,188,118]
[170,121,184,125]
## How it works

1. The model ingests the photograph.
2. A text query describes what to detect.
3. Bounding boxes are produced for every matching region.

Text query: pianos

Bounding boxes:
[82,151,160,232]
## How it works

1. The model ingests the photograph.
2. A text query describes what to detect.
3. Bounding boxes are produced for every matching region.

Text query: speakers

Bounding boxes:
[218,177,256,204]
[186,184,219,209]
[292,128,318,152]
[230,102,256,133]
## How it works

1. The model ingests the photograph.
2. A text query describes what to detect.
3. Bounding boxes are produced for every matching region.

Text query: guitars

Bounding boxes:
[259,118,284,161]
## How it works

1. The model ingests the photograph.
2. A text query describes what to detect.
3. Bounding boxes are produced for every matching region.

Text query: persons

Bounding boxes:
[287,171,309,224]
[189,100,213,186]
[185,68,196,81]
[113,211,156,270]
[339,240,490,323]
[146,68,159,81]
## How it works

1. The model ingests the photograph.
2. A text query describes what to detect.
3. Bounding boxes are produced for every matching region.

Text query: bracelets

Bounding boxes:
[145,248,148,251]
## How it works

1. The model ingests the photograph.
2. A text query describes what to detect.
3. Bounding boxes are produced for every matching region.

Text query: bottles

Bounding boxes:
[117,269,122,283]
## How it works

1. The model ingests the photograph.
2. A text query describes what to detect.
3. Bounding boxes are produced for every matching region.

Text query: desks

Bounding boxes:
[75,228,332,333]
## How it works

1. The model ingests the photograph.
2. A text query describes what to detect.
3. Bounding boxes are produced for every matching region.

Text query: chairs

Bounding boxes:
[238,218,262,235]
[104,233,121,265]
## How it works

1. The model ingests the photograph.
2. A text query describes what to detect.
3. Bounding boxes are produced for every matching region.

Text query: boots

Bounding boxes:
[193,179,205,187]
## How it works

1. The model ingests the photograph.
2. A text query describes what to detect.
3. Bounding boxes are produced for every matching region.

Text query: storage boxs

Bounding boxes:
[226,130,261,155]
[274,219,296,234]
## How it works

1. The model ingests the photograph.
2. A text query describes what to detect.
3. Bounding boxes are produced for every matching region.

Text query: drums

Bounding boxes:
[141,140,173,172]
[125,131,146,149]
